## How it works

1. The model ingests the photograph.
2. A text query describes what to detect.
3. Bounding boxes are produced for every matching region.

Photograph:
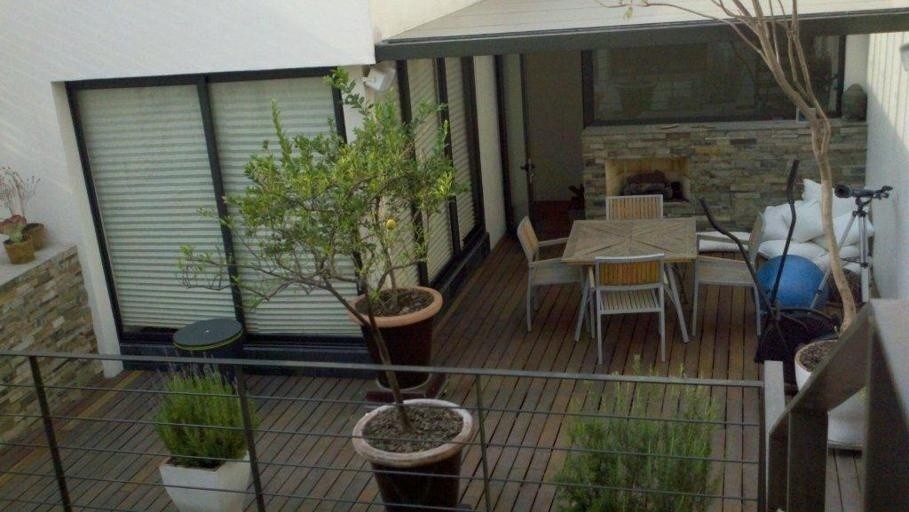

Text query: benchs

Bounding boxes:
[697,231,752,257]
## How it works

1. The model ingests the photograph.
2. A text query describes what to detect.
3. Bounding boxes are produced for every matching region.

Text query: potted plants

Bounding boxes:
[146,348,259,512]
[598,1,869,453]
[1,164,44,251]
[0,216,35,265]
[169,67,474,511]
[348,176,471,388]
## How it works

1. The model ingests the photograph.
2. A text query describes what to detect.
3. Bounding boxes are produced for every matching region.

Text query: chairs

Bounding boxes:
[517,216,590,330]
[692,211,760,337]
[605,194,663,220]
[592,253,667,363]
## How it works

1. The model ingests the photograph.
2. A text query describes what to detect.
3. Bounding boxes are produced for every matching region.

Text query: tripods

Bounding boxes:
[810,197,893,310]
